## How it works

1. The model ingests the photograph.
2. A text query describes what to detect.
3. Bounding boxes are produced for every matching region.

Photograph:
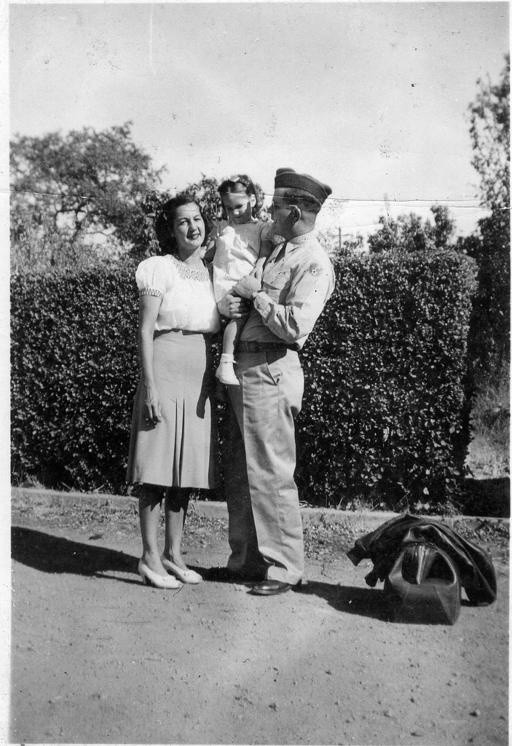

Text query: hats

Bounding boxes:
[274,168,332,204]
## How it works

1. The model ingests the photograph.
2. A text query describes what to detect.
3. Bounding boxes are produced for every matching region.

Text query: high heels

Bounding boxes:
[138,559,181,588]
[161,553,203,584]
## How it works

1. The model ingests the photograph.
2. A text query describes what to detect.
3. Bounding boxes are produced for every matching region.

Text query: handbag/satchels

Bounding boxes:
[384,543,460,624]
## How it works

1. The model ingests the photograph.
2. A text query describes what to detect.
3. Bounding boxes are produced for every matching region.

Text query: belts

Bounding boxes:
[234,341,287,351]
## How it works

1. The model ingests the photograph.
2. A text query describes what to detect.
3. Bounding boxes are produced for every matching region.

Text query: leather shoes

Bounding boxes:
[208,566,265,581]
[252,579,301,594]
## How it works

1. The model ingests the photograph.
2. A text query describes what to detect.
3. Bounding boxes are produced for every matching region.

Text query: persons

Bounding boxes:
[125,192,221,590]
[205,174,275,389]
[209,167,336,596]
